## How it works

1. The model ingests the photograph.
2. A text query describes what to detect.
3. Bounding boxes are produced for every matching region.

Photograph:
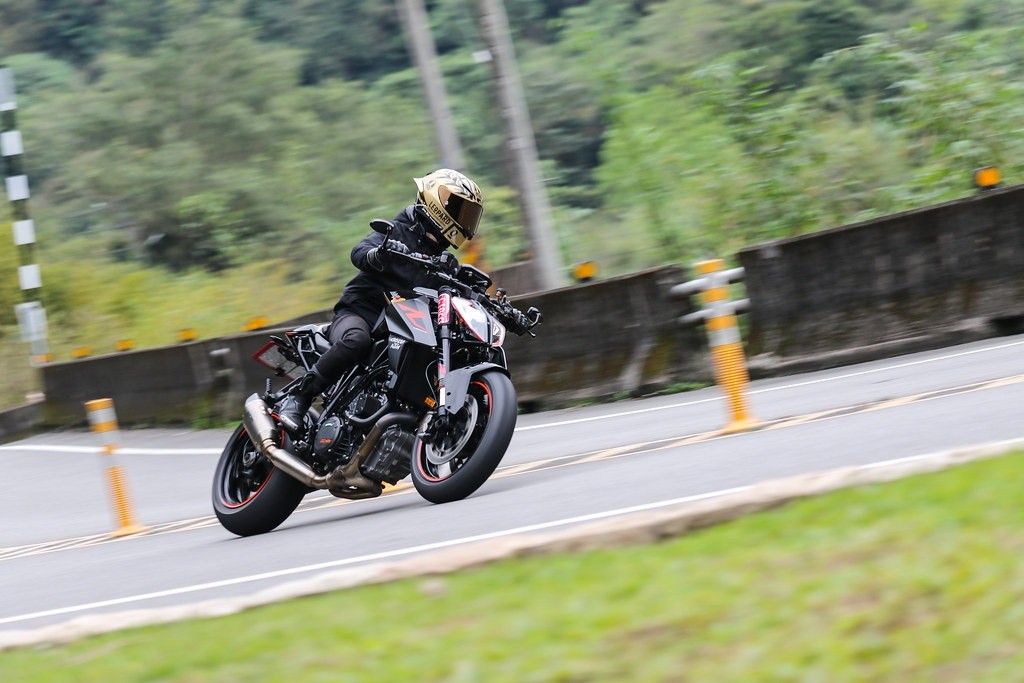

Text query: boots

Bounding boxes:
[278,363,329,432]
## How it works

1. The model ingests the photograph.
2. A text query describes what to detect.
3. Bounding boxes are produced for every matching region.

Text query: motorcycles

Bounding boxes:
[208,219,542,538]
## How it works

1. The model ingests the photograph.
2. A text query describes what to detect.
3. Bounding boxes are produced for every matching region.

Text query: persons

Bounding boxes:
[268,168,530,432]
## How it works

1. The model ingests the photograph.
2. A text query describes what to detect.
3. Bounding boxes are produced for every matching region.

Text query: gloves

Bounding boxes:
[504,307,530,337]
[367,238,410,271]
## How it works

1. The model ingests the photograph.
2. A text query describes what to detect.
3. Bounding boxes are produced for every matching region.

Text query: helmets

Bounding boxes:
[413,169,484,251]
